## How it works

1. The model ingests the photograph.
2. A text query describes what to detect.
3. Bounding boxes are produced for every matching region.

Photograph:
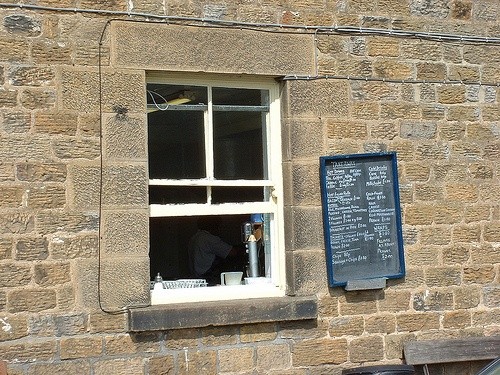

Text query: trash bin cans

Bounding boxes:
[341,364,416,375]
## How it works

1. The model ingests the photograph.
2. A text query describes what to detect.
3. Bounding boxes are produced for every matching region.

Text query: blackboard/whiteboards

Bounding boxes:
[319,150,406,288]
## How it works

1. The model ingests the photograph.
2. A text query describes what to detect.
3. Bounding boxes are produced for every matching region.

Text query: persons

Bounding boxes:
[186,217,246,283]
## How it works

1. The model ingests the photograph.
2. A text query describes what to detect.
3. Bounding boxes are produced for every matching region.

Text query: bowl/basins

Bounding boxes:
[161,279,206,290]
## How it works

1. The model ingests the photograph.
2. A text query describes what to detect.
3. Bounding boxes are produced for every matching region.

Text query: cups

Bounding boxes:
[221,272,242,286]
